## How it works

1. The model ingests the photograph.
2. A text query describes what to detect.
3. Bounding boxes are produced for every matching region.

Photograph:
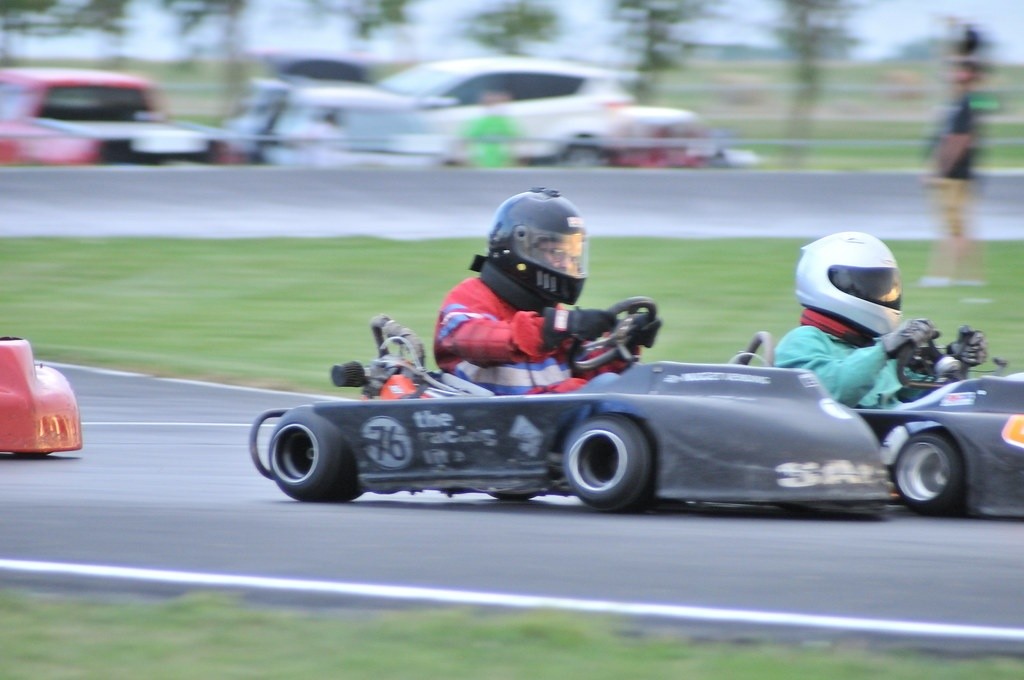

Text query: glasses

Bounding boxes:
[539,248,584,274]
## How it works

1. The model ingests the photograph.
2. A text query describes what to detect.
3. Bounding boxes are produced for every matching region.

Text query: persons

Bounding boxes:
[433,190,663,397]
[453,90,530,165]
[917,60,984,285]
[774,231,988,409]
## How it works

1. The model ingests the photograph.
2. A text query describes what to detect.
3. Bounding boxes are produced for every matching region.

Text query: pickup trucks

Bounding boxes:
[0,65,247,168]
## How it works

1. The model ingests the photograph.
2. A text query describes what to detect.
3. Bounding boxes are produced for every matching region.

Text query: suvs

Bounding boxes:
[235,50,645,169]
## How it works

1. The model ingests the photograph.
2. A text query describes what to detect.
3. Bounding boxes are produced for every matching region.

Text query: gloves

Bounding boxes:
[541,306,617,342]
[881,317,939,359]
[612,312,661,348]
[950,325,988,366]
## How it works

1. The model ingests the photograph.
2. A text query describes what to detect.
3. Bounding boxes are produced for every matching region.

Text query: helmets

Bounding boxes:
[489,187,587,304]
[795,231,903,336]
